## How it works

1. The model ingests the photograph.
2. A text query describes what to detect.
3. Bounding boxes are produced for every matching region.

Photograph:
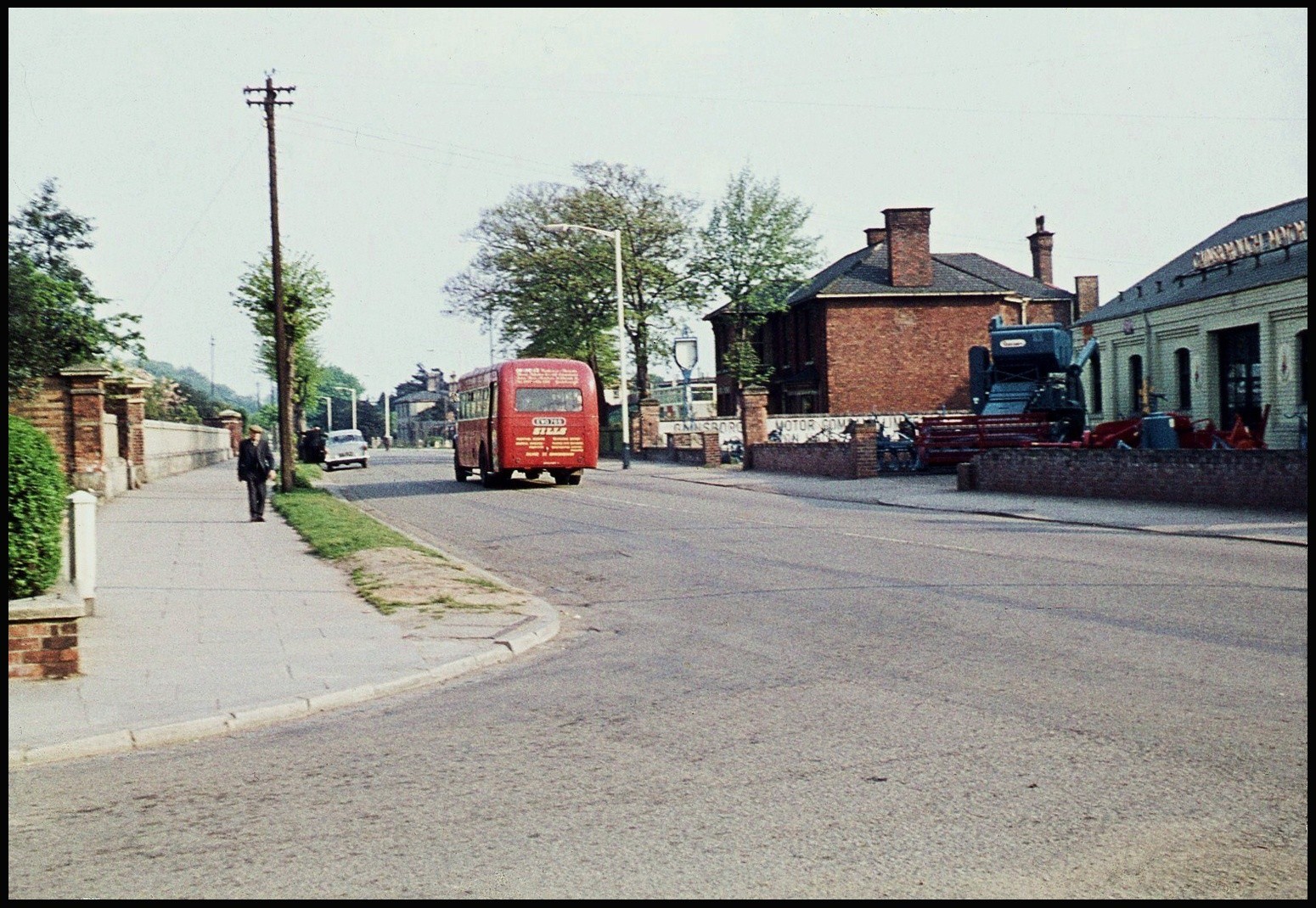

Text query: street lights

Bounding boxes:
[334,387,357,430]
[545,221,632,470]
[321,397,332,433]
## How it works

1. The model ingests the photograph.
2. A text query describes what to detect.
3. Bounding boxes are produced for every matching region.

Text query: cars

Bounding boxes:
[323,429,370,470]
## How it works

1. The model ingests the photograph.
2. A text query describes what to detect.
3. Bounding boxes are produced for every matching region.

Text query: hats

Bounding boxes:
[248,424,262,433]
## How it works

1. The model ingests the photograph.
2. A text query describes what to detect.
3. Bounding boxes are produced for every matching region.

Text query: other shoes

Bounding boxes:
[251,516,264,523]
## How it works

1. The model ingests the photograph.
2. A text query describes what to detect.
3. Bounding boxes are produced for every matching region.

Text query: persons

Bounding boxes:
[237,425,275,521]
[298,425,322,466]
[769,430,780,442]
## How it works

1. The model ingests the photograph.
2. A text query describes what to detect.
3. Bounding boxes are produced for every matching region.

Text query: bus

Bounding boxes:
[451,358,599,485]
